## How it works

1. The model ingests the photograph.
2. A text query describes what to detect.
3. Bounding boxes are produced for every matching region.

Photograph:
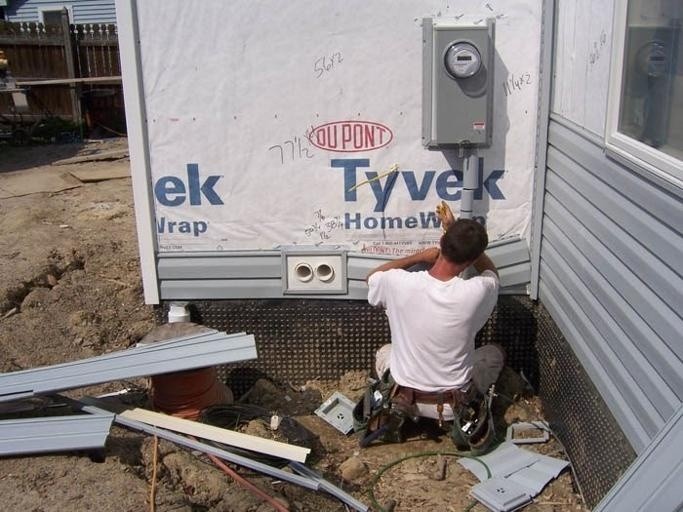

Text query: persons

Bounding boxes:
[366,201,509,423]
